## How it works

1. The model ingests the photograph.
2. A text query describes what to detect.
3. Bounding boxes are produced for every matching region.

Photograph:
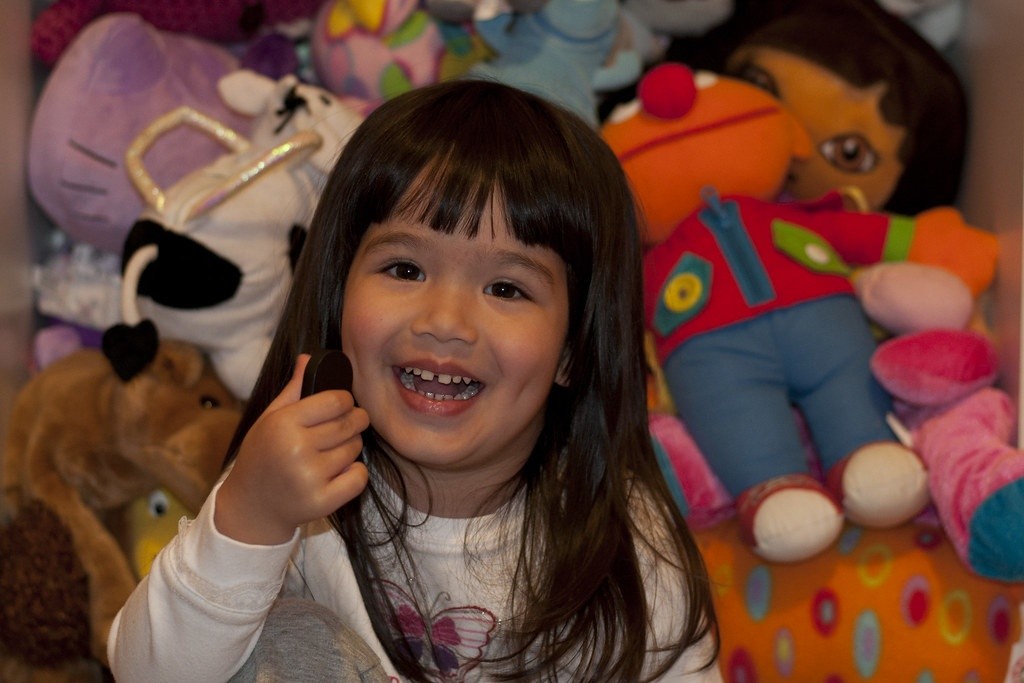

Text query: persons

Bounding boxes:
[106,81,723,683]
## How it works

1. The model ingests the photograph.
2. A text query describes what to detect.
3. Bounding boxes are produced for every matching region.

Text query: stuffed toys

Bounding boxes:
[0,0,1024,683]
